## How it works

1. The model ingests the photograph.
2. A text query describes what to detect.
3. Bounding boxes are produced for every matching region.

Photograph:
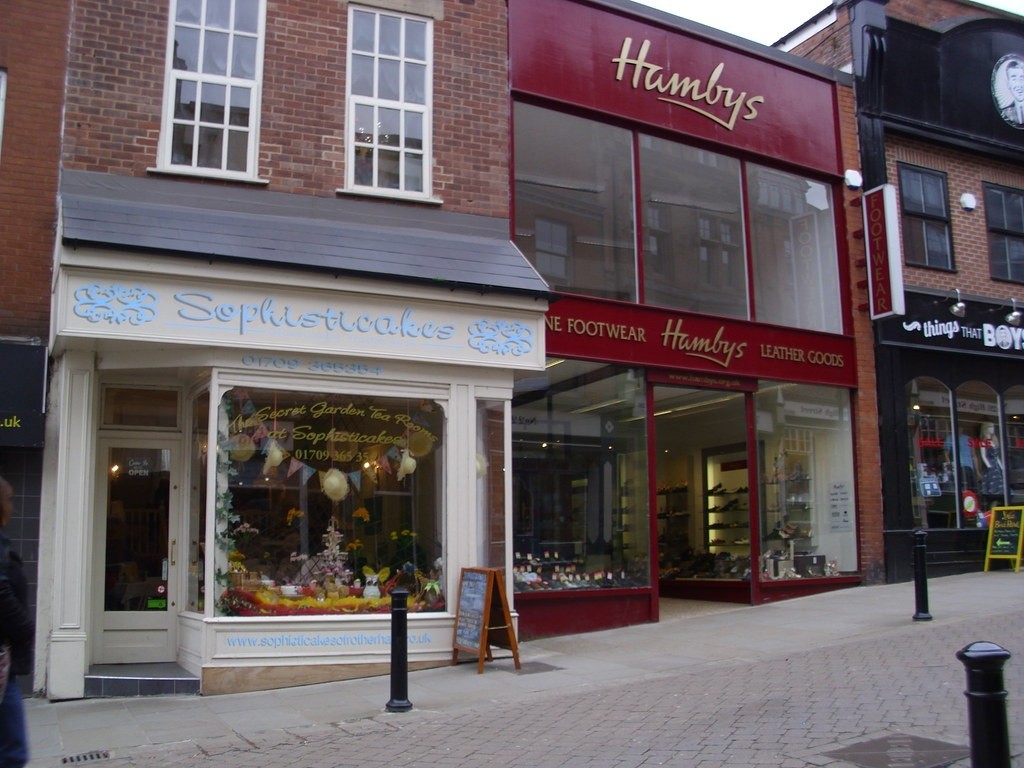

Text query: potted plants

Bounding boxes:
[362,566,389,598]
[327,584,339,599]
[315,588,325,601]
[281,576,301,595]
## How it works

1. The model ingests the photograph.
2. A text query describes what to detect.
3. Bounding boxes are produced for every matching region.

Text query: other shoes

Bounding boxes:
[708,482,751,544]
[514,572,651,594]
[717,549,802,579]
[806,567,822,578]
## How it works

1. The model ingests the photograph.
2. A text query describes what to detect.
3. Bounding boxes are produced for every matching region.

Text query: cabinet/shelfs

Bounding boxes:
[656,455,694,548]
[701,441,767,554]
[767,429,815,554]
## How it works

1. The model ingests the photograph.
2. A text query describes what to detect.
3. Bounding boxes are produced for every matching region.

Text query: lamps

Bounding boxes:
[988,298,1021,327]
[933,287,966,318]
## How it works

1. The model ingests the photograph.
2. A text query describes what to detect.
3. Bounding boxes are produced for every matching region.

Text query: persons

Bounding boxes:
[0,478,36,768]
[943,421,1004,510]
